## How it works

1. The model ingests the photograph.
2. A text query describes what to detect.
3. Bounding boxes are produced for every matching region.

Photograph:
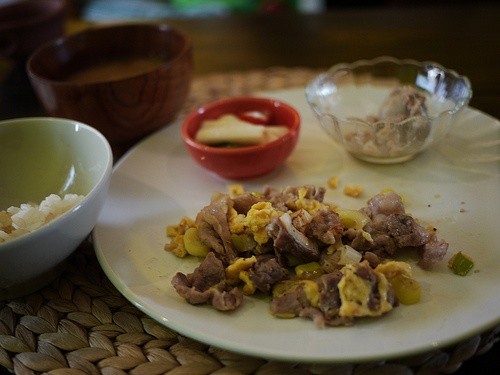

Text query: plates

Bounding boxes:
[93,86,500,361]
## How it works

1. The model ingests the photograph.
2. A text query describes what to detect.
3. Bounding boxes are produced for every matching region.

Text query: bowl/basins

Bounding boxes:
[0,117,114,287]
[27,22,194,144]
[303,56,473,164]
[182,96,301,180]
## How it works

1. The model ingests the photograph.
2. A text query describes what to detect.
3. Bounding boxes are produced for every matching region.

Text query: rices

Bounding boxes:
[0,193,86,243]
